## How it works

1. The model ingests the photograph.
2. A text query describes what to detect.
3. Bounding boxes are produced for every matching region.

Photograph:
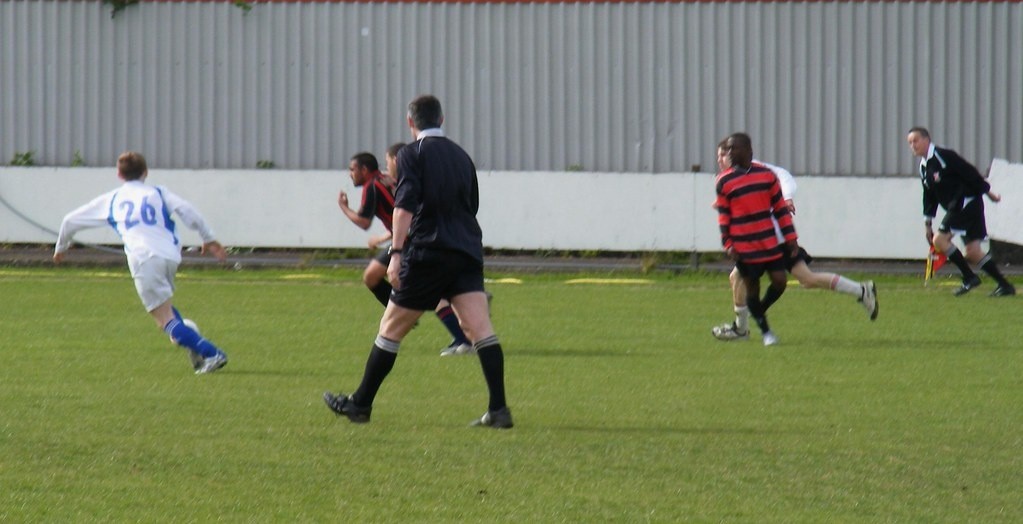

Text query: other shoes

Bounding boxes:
[763,331,776,347]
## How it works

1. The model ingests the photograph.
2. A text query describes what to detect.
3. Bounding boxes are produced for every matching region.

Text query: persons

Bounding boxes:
[340,142,494,357]
[908,126,1015,299]
[55,152,232,375]
[322,95,513,429]
[712,144,880,340]
[715,132,800,348]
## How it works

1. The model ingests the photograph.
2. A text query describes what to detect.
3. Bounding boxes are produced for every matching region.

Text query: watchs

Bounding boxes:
[388,247,403,258]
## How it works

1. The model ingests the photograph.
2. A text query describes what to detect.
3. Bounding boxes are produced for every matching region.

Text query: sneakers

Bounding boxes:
[190,351,202,369]
[856,280,878,321]
[440,338,473,357]
[712,322,750,341]
[990,284,1016,296]
[471,408,513,429]
[953,275,982,296]
[325,392,372,423]
[194,349,227,374]
